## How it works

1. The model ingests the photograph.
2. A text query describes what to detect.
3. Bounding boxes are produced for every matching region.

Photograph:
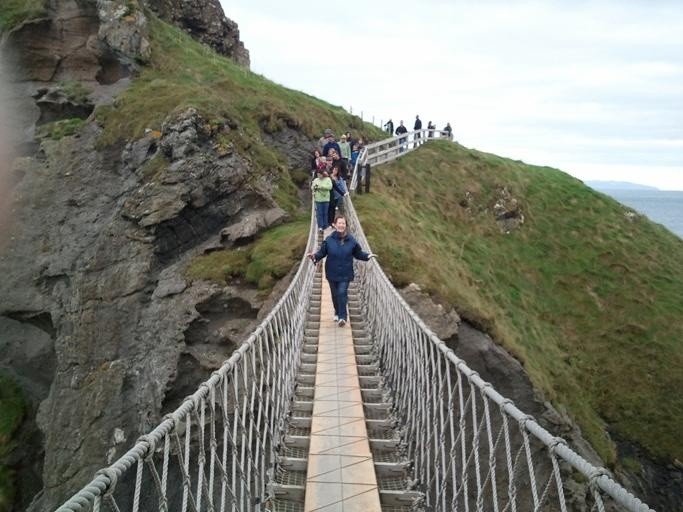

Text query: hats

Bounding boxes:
[324,129,332,134]
[340,134,347,139]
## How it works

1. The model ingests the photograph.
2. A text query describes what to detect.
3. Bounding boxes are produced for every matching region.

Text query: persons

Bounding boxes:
[442,121,451,138]
[412,115,422,148]
[427,120,435,141]
[309,127,365,234]
[305,216,379,329]
[383,118,393,135]
[394,120,407,153]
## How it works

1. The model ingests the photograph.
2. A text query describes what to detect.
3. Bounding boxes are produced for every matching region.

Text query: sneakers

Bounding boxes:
[319,228,322,233]
[338,318,345,327]
[327,226,332,230]
[334,315,338,321]
